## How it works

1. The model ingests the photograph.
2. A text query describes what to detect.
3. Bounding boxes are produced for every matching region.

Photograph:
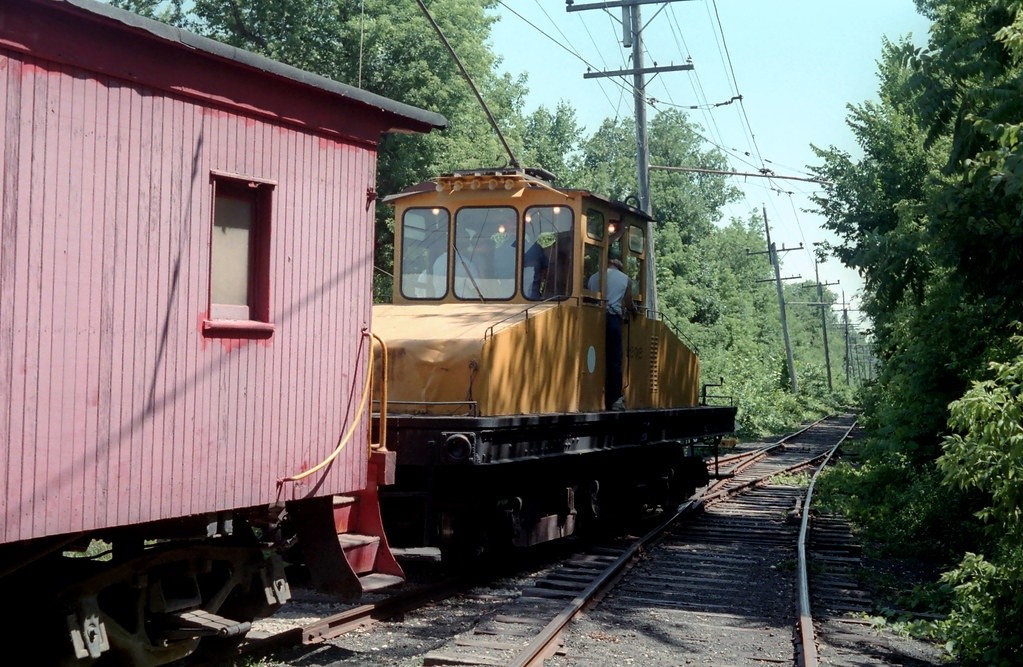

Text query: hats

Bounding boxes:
[608,246,623,260]
[537,232,558,248]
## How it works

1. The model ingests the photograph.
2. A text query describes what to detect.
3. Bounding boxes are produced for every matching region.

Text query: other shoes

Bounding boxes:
[611,396,625,412]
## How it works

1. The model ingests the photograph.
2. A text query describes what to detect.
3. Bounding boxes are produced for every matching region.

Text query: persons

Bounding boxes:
[413,210,571,301]
[587,253,639,411]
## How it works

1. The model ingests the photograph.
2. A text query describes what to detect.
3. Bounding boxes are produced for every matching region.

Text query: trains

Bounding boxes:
[1,0,740,667]
